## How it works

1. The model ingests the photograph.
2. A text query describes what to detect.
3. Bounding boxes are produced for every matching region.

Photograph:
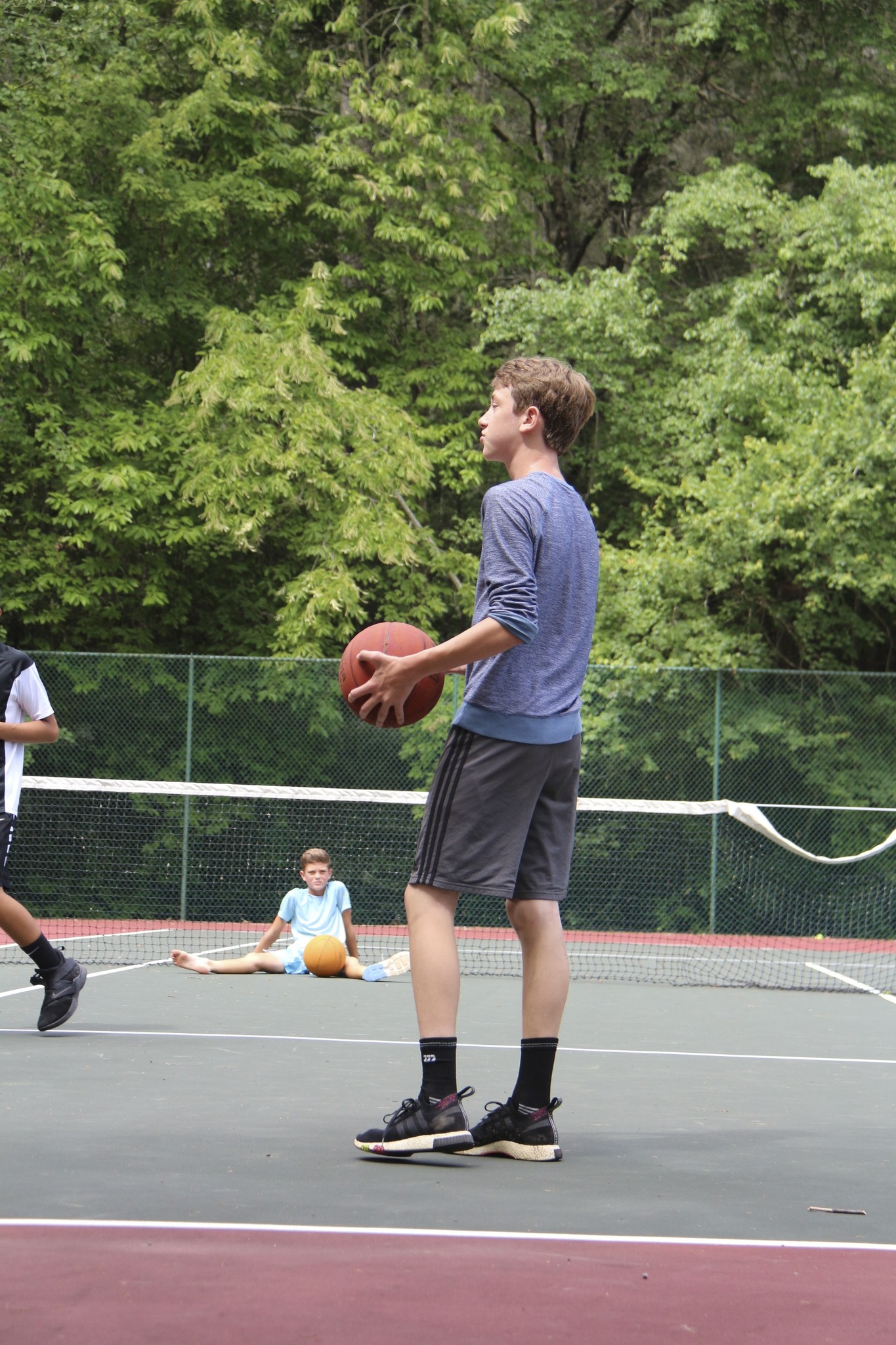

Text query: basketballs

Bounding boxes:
[338,621,445,728]
[303,934,346,978]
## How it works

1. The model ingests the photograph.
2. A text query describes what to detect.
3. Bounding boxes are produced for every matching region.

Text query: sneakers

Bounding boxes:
[354,1086,474,1157]
[454,1097,562,1161]
[30,948,87,1031]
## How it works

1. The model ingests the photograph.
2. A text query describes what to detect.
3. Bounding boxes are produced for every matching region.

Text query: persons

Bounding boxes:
[171,848,411,982]
[0,643,86,1031]
[346,357,598,1162]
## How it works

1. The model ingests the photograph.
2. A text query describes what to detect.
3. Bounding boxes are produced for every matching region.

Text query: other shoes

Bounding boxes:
[362,951,411,981]
